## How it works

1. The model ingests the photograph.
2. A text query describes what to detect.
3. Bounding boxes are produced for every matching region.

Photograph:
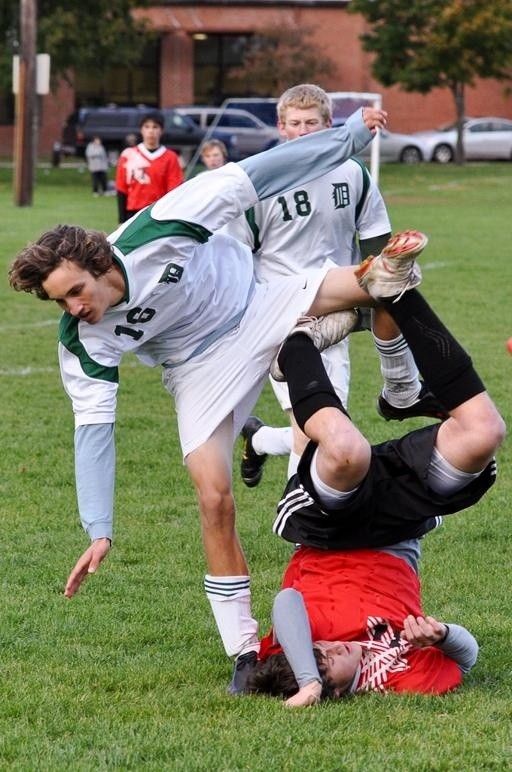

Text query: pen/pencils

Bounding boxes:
[170,109,287,159]
[62,104,239,169]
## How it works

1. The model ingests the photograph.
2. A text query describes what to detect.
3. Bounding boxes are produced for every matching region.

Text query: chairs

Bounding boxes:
[354,230,428,302]
[270,307,359,382]
[377,380,450,421]
[241,416,268,486]
[231,651,258,691]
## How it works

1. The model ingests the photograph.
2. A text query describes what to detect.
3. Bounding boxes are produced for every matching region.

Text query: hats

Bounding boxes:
[412,115,512,167]
[330,119,428,166]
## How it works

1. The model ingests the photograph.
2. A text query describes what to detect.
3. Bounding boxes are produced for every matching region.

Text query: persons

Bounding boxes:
[201,139,229,170]
[120,132,138,151]
[84,135,112,198]
[238,83,393,490]
[115,111,183,225]
[247,229,508,709]
[9,104,448,698]
